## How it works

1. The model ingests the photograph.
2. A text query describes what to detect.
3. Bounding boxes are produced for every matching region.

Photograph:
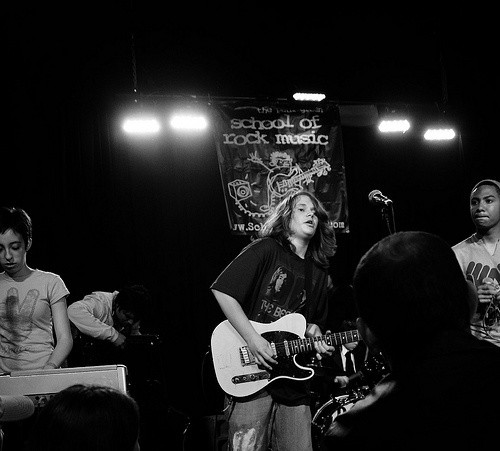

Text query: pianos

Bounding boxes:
[0,364,130,408]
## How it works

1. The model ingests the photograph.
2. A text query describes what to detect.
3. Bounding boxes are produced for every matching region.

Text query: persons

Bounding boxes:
[208,191,338,451]
[319,231,500,451]
[328,319,365,384]
[0,207,153,451]
[452,178,500,347]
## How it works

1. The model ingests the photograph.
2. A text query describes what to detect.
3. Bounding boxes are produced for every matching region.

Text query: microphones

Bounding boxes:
[482,277,494,295]
[368,190,393,207]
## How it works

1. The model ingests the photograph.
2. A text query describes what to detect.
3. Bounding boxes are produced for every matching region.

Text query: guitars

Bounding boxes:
[210,313,364,402]
